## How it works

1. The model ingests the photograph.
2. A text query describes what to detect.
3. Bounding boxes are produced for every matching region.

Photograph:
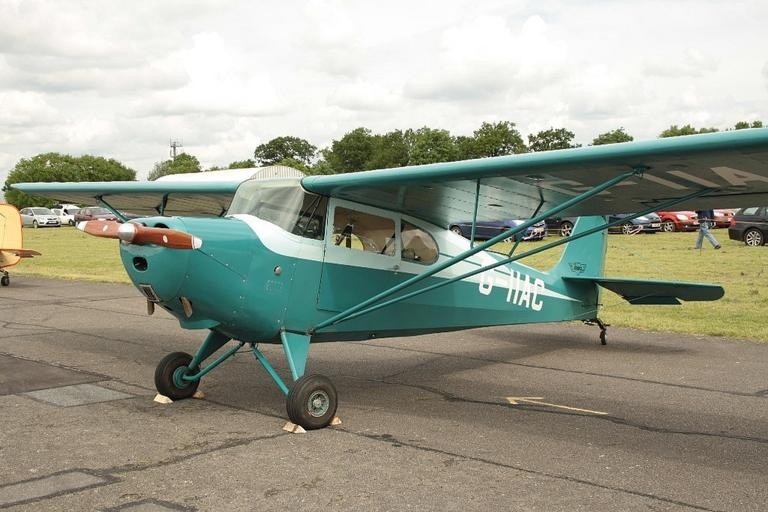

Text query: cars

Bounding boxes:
[19,202,126,229]
[727,206,768,248]
[446,207,740,243]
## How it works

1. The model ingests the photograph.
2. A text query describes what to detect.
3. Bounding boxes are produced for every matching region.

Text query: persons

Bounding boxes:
[693,209,721,250]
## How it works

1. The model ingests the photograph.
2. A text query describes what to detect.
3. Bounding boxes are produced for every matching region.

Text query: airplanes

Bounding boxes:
[1,203,42,287]
[6,124,768,435]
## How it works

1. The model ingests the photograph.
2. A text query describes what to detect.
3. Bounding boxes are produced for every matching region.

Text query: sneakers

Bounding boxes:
[715,245,721,250]
